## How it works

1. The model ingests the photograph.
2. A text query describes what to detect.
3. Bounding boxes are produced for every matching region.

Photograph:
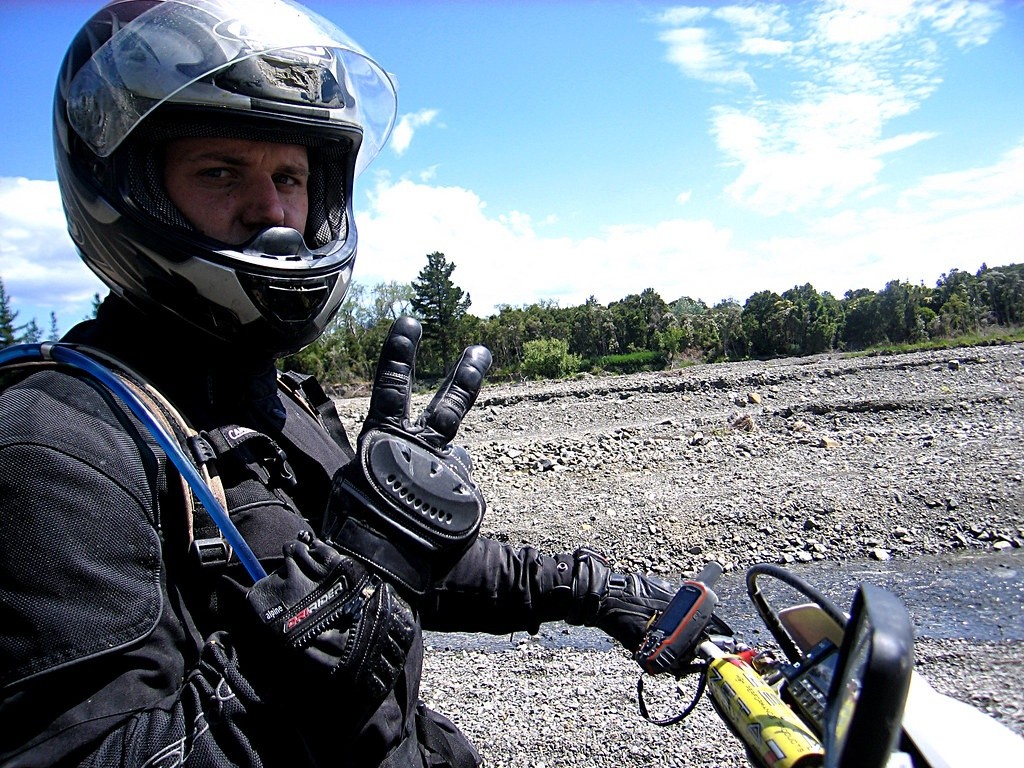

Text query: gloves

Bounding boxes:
[245,314,494,741]
[570,545,731,678]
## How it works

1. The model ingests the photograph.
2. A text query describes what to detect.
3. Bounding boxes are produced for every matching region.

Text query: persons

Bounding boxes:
[0,1,735,767]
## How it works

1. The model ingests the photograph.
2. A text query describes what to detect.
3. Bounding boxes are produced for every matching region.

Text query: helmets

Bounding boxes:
[53,0,398,361]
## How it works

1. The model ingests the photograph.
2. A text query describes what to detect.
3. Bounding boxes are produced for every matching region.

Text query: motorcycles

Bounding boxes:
[637,563,1024,768]
